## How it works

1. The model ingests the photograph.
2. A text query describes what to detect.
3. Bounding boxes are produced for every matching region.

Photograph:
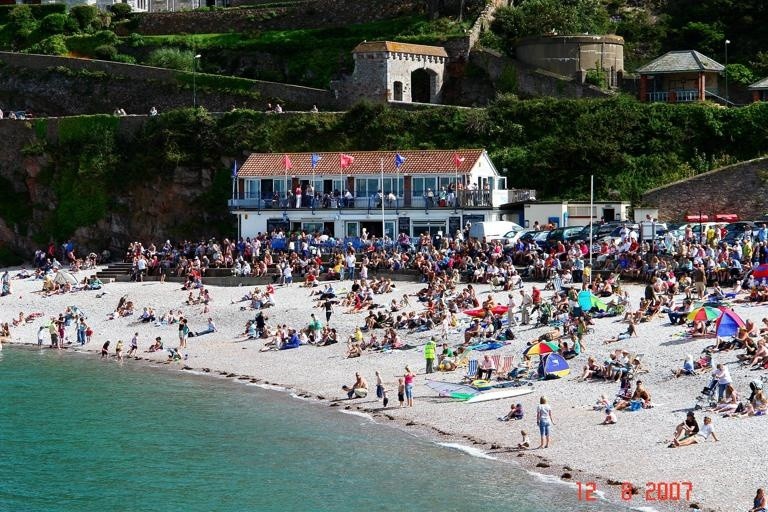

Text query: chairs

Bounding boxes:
[628,297,665,322]
[585,348,644,383]
[466,355,538,383]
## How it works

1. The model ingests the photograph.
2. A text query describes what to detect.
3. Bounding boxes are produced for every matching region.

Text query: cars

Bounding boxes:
[14,110,33,117]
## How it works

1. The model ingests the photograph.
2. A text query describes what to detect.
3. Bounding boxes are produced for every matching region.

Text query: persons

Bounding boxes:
[112,106,128,116]
[274,103,283,113]
[149,106,158,116]
[265,103,275,112]
[310,104,319,112]
[0,105,34,120]
[748,487,768,512]
[230,104,238,112]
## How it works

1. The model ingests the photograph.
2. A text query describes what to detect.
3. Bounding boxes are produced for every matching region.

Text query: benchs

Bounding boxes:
[512,256,625,283]
[90,261,384,284]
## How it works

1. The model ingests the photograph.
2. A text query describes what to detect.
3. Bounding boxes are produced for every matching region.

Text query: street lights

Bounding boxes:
[723,38,731,100]
[192,53,201,108]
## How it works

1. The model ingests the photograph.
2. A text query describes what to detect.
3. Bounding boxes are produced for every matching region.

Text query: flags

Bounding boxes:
[231,160,240,177]
[454,151,466,168]
[282,154,292,170]
[396,151,406,170]
[311,152,322,170]
[340,153,355,169]
